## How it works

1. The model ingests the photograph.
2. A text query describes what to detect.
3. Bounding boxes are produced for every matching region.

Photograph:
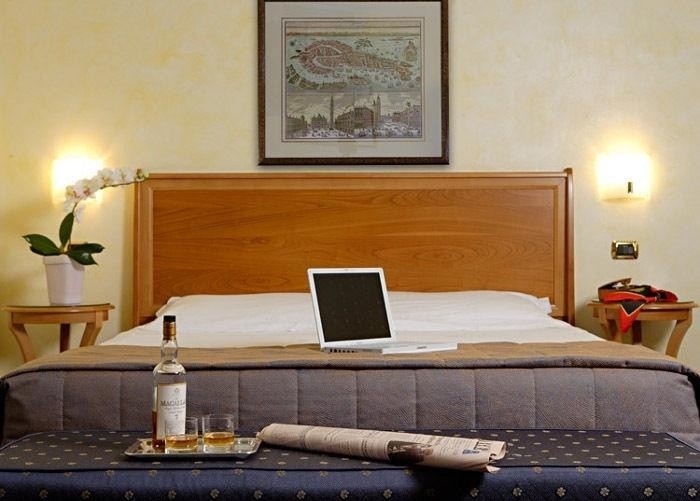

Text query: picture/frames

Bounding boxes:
[257,1,449,166]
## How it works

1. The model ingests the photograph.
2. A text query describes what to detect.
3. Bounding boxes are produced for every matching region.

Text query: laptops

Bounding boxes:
[308,266,458,354]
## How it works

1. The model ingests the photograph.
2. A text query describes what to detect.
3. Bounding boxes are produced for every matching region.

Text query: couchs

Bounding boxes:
[586,299,700,359]
[2,301,116,364]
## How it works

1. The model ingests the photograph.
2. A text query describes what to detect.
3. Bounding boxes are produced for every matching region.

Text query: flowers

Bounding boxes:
[21,167,148,267]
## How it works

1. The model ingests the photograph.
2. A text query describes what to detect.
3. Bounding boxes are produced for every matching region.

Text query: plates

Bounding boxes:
[123,437,262,460]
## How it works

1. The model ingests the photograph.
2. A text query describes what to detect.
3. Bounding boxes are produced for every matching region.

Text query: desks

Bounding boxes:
[0,427,700,500]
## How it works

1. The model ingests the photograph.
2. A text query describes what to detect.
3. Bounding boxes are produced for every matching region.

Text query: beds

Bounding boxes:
[3,167,700,438]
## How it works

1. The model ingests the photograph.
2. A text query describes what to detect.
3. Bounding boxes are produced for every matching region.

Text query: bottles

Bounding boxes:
[152,315,187,450]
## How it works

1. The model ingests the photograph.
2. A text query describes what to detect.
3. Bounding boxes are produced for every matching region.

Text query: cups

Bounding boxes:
[201,414,235,454]
[163,416,199,455]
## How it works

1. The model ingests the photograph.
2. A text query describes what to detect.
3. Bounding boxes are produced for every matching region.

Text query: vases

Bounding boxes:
[39,253,86,308]
